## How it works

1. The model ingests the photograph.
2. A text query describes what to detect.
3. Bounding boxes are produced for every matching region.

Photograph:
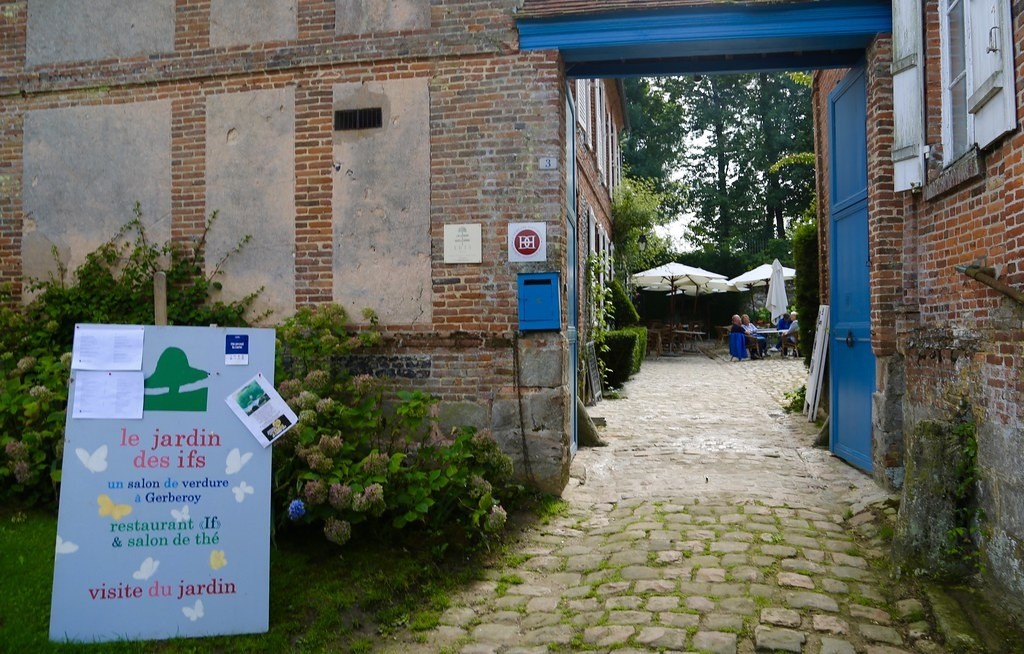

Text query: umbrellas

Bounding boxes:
[633,260,762,352]
[765,257,789,329]
[730,262,796,295]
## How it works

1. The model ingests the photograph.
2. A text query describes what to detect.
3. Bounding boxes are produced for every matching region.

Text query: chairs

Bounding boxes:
[646,319,798,361]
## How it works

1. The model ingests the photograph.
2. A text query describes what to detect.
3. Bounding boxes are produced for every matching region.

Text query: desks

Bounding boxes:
[672,330,706,353]
[757,329,789,352]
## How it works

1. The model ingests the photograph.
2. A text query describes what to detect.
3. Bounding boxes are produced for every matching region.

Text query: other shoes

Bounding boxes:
[751,353,760,360]
[764,351,771,357]
[769,347,778,352]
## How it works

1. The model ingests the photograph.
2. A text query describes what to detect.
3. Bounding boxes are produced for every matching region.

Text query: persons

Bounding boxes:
[769,310,799,356]
[740,314,772,357]
[731,314,762,360]
[775,312,793,339]
